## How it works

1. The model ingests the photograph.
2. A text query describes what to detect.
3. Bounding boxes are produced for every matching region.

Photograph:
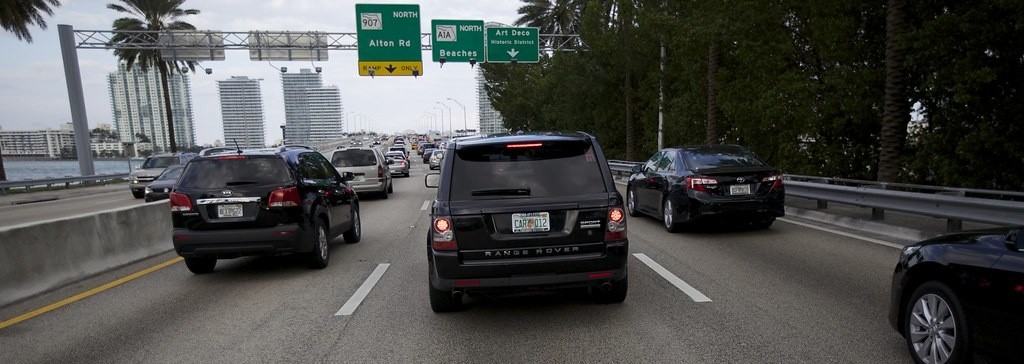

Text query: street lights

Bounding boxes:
[416,98,467,138]
[346,111,374,135]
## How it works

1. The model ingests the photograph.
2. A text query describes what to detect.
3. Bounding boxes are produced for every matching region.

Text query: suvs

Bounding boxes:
[331,145,394,200]
[426,130,629,314]
[128,152,198,199]
[169,145,361,275]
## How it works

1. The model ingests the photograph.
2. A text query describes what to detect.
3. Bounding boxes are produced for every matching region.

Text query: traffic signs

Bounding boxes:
[356,3,424,79]
[431,22,486,65]
[485,27,541,63]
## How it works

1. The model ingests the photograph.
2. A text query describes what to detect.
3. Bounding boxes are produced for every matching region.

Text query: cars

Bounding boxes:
[423,148,436,164]
[350,134,447,156]
[145,164,187,203]
[626,145,786,234]
[429,149,446,170]
[889,225,1024,364]
[384,151,410,177]
